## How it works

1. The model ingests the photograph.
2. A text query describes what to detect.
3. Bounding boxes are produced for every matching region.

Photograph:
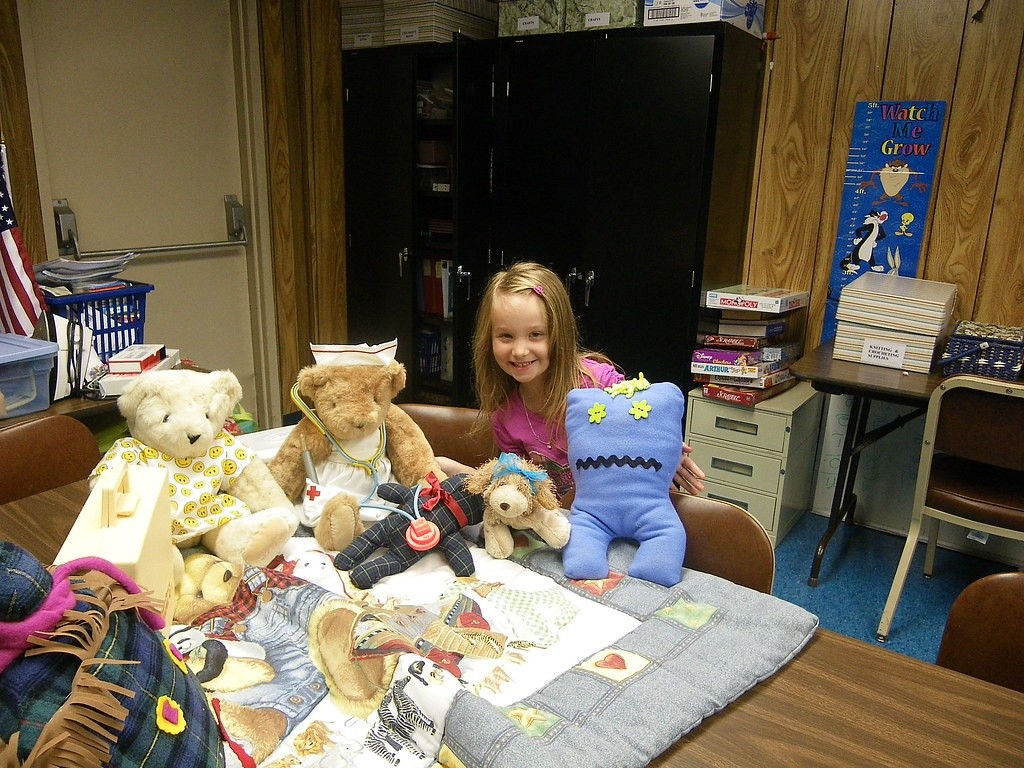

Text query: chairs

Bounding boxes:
[0,373,1024,694]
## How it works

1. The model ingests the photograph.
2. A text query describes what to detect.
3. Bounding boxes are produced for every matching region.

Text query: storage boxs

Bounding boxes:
[341,0,766,50]
[690,271,1023,406]
[38,278,155,391]
[0,333,59,422]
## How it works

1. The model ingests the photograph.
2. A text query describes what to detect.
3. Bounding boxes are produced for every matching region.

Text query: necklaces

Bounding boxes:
[519,387,555,449]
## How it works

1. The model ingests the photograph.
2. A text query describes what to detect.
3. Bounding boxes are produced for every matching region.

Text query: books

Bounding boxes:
[31,252,141,284]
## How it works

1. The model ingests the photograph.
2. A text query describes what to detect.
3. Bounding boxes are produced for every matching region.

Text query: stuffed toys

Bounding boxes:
[561,372,686,586]
[465,452,572,558]
[86,370,299,586]
[334,474,486,590]
[269,338,449,549]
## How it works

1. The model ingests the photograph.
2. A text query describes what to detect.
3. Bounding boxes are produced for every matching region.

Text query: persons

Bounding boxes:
[434,263,705,508]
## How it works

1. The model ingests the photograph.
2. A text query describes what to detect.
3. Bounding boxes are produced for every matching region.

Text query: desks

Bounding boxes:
[787,342,1024,586]
[0,485,1024,768]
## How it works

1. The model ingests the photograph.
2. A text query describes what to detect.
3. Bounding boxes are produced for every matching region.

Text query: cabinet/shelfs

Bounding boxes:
[343,22,824,552]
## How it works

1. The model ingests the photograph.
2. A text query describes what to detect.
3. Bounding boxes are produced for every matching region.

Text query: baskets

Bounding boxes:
[939,319,1024,383]
[46,277,154,362]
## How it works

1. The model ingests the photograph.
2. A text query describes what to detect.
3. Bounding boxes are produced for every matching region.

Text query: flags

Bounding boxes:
[0,148,48,337]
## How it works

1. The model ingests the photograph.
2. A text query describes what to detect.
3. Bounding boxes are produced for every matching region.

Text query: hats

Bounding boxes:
[0,540,54,622]
